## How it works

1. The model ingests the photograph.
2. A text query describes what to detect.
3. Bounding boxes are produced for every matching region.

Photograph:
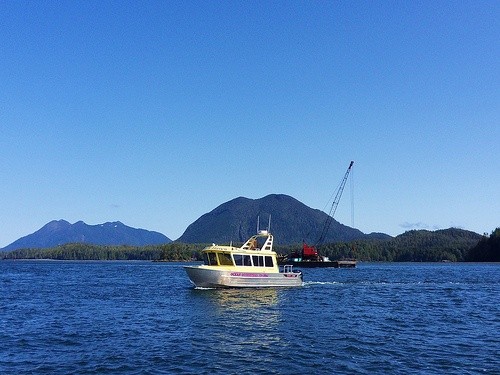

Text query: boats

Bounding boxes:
[277,257,356,271]
[182,230,303,289]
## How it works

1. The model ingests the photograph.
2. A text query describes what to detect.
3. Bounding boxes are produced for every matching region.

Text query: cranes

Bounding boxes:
[289,161,354,256]
